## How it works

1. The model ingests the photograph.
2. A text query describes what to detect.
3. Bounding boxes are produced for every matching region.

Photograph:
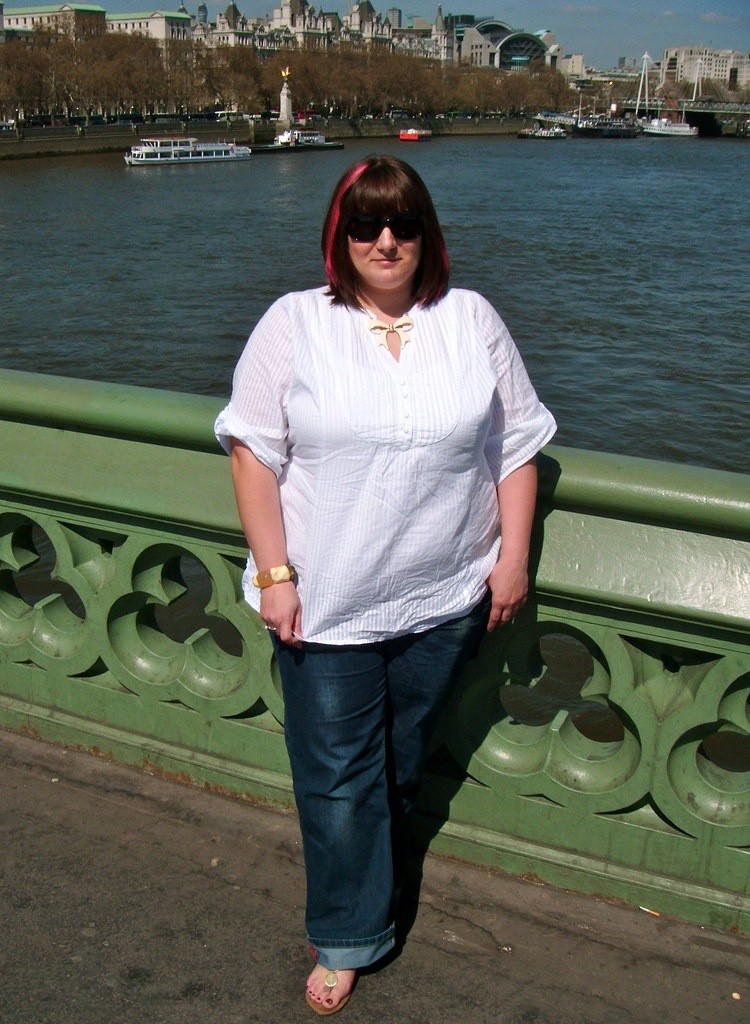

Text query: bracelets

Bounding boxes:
[252,564,299,589]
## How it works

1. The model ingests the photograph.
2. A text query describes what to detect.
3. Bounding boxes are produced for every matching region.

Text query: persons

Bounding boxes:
[214,154,559,1016]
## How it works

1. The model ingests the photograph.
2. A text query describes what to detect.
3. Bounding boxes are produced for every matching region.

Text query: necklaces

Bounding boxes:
[355,292,414,351]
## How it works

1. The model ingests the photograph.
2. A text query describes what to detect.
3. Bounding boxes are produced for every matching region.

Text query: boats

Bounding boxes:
[399,129,432,142]
[516,50,702,140]
[249,128,345,154]
[124,136,253,166]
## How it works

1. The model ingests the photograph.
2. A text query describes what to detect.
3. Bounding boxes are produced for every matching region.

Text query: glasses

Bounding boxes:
[344,210,421,242]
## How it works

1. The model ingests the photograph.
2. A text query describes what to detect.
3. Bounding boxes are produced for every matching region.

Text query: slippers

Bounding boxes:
[304,962,357,1015]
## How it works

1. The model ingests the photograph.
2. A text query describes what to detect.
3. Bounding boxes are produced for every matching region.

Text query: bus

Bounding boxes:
[445,112,464,121]
[29,113,205,127]
[388,109,410,120]
[480,111,501,119]
[214,110,322,123]
